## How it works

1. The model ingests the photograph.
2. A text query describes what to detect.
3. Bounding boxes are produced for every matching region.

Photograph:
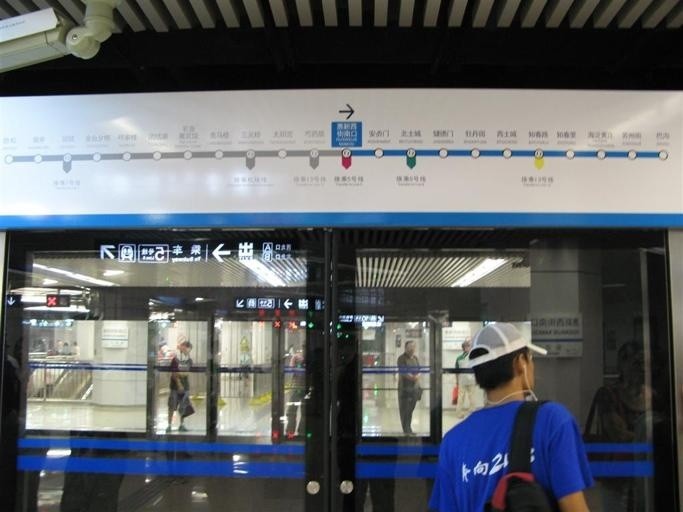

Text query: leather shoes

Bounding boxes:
[166,428,174,432]
[178,426,188,432]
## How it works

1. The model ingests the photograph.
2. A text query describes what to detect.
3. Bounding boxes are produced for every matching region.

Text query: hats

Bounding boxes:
[456,322,549,368]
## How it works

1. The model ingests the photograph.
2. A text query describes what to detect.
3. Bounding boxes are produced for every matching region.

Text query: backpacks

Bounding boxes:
[484,401,558,512]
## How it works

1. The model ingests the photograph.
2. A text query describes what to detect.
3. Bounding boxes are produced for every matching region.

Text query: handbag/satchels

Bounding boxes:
[407,382,424,403]
[452,385,457,404]
[178,398,195,417]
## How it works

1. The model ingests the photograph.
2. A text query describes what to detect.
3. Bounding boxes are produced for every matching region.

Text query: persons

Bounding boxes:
[454,340,477,417]
[396,341,424,437]
[584,339,656,511]
[165,341,192,433]
[426,322,595,512]
[33,337,78,356]
[285,343,304,434]
[159,336,166,349]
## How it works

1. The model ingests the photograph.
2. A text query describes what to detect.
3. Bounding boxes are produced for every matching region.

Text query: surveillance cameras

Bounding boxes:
[0,9,74,74]
